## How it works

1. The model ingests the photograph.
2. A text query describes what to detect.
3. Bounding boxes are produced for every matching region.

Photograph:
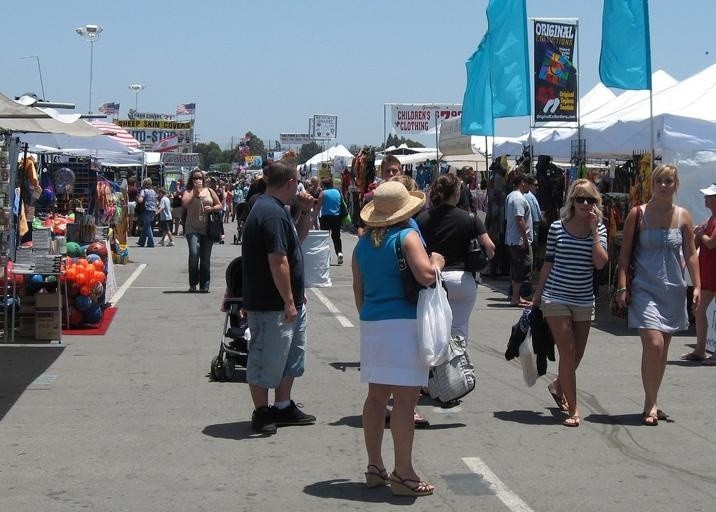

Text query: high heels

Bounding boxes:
[365,465,389,488]
[390,472,434,496]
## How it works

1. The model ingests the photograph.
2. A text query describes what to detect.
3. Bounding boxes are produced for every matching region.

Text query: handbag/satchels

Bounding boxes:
[609,265,635,316]
[302,230,331,289]
[207,213,224,241]
[340,202,348,219]
[416,288,453,368]
[466,234,490,271]
[427,335,475,403]
[341,213,352,227]
[135,202,145,213]
[403,251,448,305]
[172,195,181,208]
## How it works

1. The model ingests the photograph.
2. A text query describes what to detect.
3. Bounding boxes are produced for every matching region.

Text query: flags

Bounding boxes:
[112,104,119,115]
[176,103,195,115]
[99,102,114,115]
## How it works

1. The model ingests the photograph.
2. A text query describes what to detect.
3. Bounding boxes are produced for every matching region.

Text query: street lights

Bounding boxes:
[76,23,103,116]
[128,80,145,134]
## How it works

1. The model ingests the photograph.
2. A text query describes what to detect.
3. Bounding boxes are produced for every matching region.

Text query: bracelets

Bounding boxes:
[697,230,703,236]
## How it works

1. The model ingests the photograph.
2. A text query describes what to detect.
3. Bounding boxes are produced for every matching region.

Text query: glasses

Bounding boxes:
[575,197,596,204]
[191,177,203,181]
[534,183,539,187]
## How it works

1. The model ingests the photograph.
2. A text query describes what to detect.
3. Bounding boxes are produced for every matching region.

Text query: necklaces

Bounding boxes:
[650,201,675,222]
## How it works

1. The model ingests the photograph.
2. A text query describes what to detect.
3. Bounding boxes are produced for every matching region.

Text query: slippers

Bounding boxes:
[513,303,531,306]
[519,299,533,304]
[700,357,716,364]
[641,413,658,426]
[548,385,568,410]
[682,352,703,361]
[561,413,579,426]
[658,410,669,419]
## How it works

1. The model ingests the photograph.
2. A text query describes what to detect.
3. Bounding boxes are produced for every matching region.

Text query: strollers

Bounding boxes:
[210,256,265,380]
[232,200,250,245]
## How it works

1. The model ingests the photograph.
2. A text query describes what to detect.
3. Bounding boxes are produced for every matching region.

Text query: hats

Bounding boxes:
[359,182,426,226]
[380,176,418,193]
[699,184,716,196]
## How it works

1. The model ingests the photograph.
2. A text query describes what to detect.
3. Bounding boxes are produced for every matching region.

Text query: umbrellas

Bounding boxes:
[88,117,141,150]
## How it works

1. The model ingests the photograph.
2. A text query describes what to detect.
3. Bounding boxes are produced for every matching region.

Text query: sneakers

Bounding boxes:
[338,253,343,264]
[272,400,316,426]
[157,240,165,246]
[168,242,176,245]
[251,406,276,434]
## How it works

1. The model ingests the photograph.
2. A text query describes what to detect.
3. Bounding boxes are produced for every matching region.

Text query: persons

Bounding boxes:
[351,180,445,497]
[240,160,319,435]
[529,179,609,428]
[294,171,379,265]
[19,146,275,248]
[228,301,254,354]
[677,181,716,365]
[180,170,221,293]
[417,175,496,410]
[380,153,624,308]
[613,164,702,427]
[365,172,440,430]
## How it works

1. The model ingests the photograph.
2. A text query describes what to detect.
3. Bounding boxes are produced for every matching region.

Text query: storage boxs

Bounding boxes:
[34,293,62,340]
